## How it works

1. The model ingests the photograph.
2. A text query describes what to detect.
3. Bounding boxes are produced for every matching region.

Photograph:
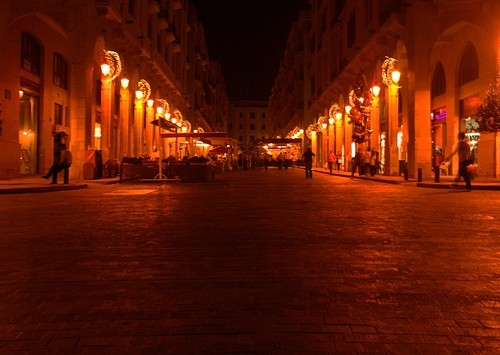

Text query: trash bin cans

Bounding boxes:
[398,160,405,176]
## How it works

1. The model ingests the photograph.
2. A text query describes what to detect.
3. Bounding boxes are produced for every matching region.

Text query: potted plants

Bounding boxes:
[120,155,217,184]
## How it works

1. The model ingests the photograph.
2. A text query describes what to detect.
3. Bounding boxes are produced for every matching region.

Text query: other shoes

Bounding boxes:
[50,182,57,184]
[42,176,49,179]
[462,188,471,192]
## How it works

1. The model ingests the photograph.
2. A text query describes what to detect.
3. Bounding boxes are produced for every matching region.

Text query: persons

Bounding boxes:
[277,152,295,169]
[432,147,441,175]
[238,156,247,169]
[355,146,379,176]
[251,160,256,169]
[302,148,316,179]
[328,151,336,172]
[448,132,473,192]
[264,159,269,170]
[42,144,72,184]
[336,151,342,171]
